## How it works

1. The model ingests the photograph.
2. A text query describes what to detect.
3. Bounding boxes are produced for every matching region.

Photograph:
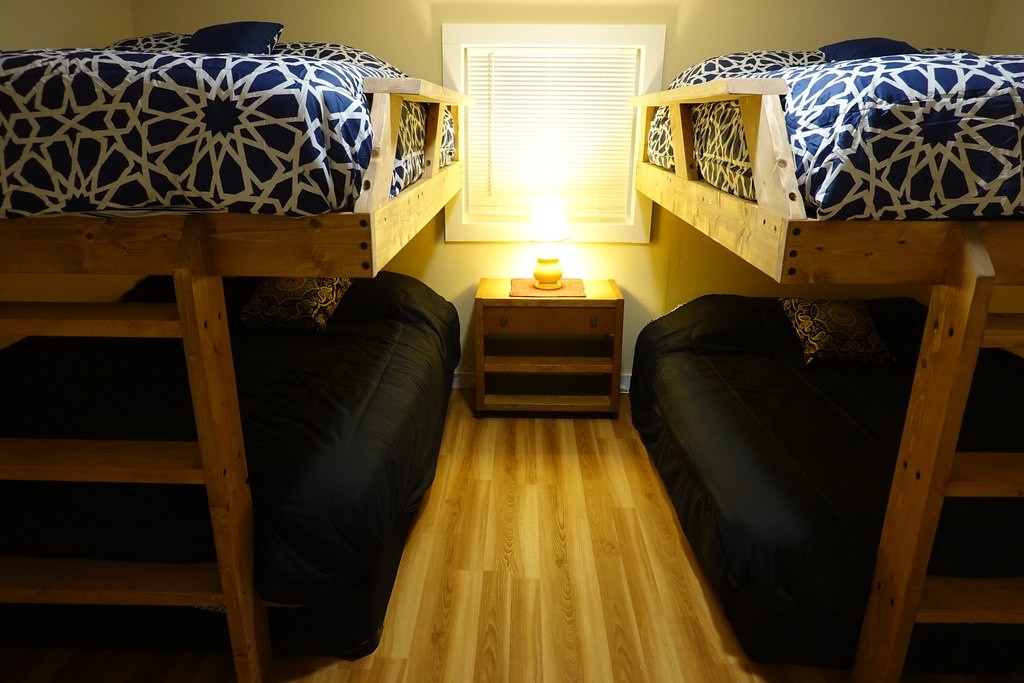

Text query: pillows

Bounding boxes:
[106,21,402,73]
[778,297,886,365]
[669,38,981,90]
[239,277,351,328]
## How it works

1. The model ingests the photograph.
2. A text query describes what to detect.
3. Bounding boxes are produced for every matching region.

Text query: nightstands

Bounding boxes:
[474,278,625,419]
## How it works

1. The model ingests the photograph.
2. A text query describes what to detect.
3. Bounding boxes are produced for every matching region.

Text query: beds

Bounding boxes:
[0,47,472,683]
[630,53,1024,683]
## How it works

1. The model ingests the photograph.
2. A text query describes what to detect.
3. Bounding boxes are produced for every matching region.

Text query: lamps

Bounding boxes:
[526,217,575,290]
